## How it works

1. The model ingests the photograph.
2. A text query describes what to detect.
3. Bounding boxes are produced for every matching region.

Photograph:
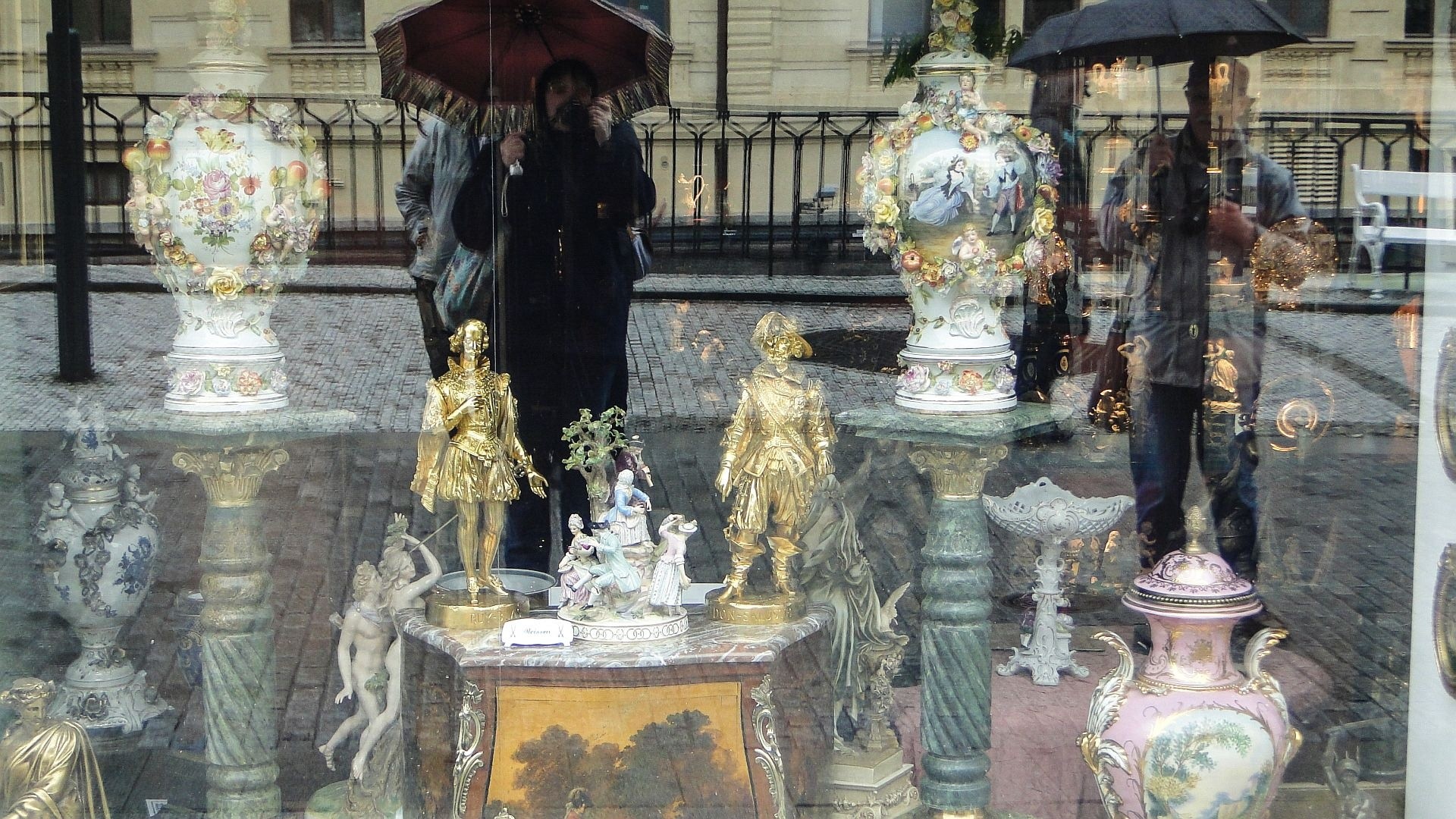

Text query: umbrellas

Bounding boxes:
[370,0,675,217]
[1009,0,1312,175]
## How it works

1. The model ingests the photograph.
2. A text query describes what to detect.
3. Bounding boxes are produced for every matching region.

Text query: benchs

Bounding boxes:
[1347,163,1456,300]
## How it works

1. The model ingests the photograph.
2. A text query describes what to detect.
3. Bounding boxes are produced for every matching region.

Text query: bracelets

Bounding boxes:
[1253,224,1266,242]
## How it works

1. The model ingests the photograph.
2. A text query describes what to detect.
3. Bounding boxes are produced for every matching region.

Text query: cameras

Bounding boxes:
[554,96,597,161]
[1159,175,1241,239]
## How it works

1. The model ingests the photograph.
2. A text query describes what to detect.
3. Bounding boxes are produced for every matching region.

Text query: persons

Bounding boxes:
[316,321,550,779]
[557,313,842,617]
[1096,57,1309,639]
[0,397,156,819]
[1093,329,1302,590]
[394,59,656,610]
[947,72,993,143]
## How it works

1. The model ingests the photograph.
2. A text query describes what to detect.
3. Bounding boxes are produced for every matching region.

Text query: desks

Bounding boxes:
[395,605,836,818]
